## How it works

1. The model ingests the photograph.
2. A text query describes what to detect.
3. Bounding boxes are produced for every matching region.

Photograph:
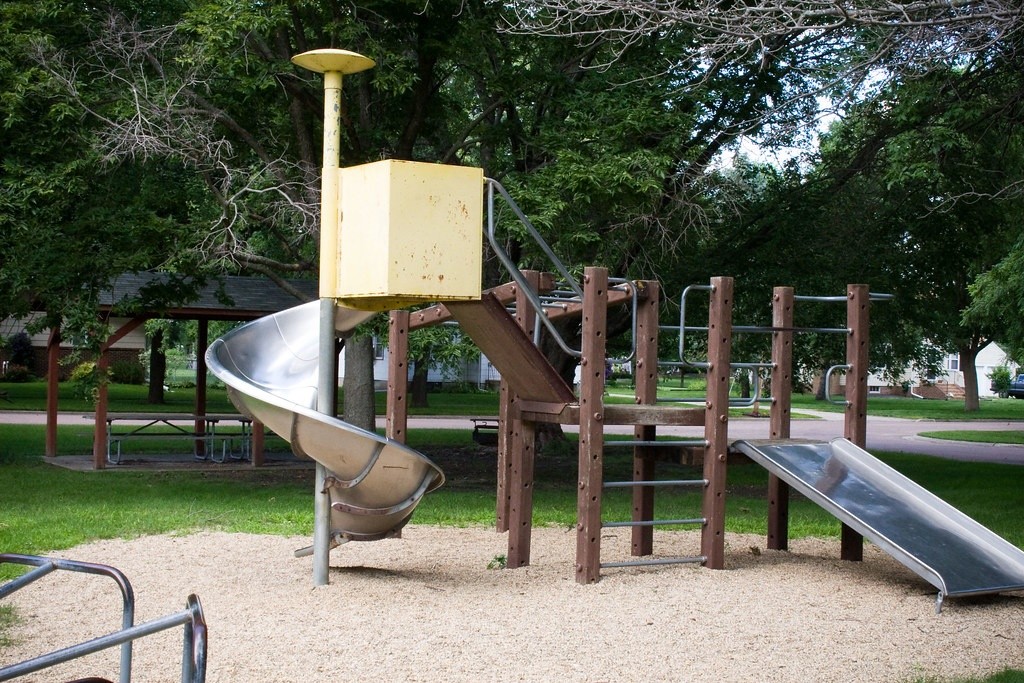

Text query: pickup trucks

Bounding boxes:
[989,374,1024,399]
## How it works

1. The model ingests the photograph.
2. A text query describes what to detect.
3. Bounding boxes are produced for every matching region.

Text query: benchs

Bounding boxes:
[90,433,278,464]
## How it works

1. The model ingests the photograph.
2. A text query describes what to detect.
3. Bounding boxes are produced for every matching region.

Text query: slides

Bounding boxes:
[202,297,448,543]
[730,436,1024,598]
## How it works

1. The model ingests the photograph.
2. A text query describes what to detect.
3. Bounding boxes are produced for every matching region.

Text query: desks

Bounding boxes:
[81,413,254,465]
